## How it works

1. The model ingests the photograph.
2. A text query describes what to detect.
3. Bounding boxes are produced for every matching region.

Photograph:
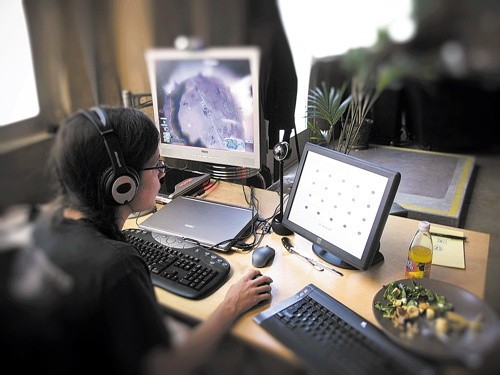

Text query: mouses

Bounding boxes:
[248,245,276,267]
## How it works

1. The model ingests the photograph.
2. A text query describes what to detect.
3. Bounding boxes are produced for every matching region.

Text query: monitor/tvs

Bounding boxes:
[281,142,401,269]
[144,46,263,169]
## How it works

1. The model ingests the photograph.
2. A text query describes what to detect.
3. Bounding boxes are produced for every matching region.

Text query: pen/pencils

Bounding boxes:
[428,233,466,241]
[313,260,343,277]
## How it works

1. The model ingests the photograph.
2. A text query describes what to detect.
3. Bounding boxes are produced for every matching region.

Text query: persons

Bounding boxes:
[6,107,273,374]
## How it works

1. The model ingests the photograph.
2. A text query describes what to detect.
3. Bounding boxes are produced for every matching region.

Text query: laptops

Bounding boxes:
[138,194,258,252]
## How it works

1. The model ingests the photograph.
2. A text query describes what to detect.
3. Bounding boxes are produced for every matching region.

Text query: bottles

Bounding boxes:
[405,220,434,281]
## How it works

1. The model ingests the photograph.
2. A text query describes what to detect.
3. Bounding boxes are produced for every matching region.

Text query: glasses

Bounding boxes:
[134,159,167,173]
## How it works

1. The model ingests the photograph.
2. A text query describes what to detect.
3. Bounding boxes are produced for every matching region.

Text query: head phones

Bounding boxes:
[80,105,139,204]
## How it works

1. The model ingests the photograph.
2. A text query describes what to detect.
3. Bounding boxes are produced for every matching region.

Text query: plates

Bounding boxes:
[372,278,500,363]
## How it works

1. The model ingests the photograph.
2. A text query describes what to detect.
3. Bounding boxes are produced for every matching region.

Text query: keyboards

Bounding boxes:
[254,285,439,373]
[100,227,231,301]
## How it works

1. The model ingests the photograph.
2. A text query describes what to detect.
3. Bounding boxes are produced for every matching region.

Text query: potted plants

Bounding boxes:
[344,74,376,150]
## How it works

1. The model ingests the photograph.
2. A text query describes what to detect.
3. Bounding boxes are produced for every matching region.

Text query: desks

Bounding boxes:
[120,168,491,375]
